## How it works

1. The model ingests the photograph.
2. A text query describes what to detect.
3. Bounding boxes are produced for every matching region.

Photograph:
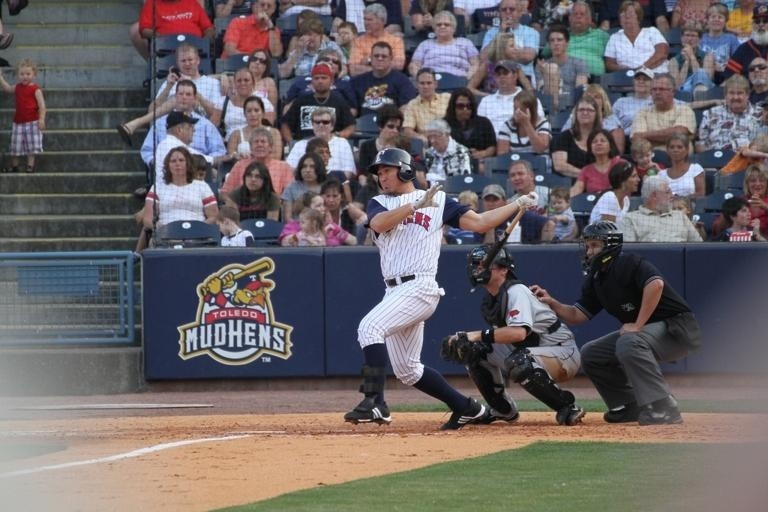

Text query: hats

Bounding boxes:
[493,59,518,72]
[481,184,506,199]
[167,110,198,128]
[634,67,654,79]
[752,5,768,20]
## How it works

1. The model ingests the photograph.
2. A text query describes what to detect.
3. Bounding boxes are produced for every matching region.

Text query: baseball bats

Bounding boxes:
[482,206,527,267]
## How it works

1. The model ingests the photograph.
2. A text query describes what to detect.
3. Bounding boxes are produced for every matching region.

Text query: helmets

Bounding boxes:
[582,221,623,280]
[467,243,518,293]
[367,147,416,189]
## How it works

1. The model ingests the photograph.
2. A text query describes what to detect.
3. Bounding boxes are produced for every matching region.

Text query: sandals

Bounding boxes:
[2,164,34,173]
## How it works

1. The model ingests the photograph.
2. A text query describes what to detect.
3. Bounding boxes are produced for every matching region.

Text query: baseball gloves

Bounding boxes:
[439,330,493,363]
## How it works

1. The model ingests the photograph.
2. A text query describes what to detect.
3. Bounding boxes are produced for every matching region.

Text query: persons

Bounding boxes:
[439,242,586,426]
[343,145,540,430]
[0,55,48,171]
[114,1,766,266]
[527,219,706,426]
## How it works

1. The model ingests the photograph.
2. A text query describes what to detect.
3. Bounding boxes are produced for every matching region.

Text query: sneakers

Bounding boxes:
[486,407,520,424]
[8,0,27,15]
[124,254,141,266]
[343,397,393,425]
[565,404,585,426]
[134,187,147,196]
[604,406,643,422]
[440,397,488,430]
[638,405,683,426]
[0,32,14,49]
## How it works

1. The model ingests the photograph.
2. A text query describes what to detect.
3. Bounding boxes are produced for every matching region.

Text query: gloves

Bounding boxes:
[516,191,539,209]
[412,182,444,209]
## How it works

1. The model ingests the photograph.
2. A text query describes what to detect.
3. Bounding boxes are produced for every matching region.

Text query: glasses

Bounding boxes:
[387,124,401,131]
[501,6,516,12]
[577,108,595,113]
[456,103,472,110]
[753,18,768,24]
[748,64,767,72]
[435,22,452,27]
[374,53,389,58]
[316,56,341,65]
[313,119,332,125]
[250,57,268,64]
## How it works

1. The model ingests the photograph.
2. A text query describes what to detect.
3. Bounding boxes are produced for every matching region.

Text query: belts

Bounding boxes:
[387,275,414,287]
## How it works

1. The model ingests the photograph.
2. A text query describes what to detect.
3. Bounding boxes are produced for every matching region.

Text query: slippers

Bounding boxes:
[116,123,133,146]
